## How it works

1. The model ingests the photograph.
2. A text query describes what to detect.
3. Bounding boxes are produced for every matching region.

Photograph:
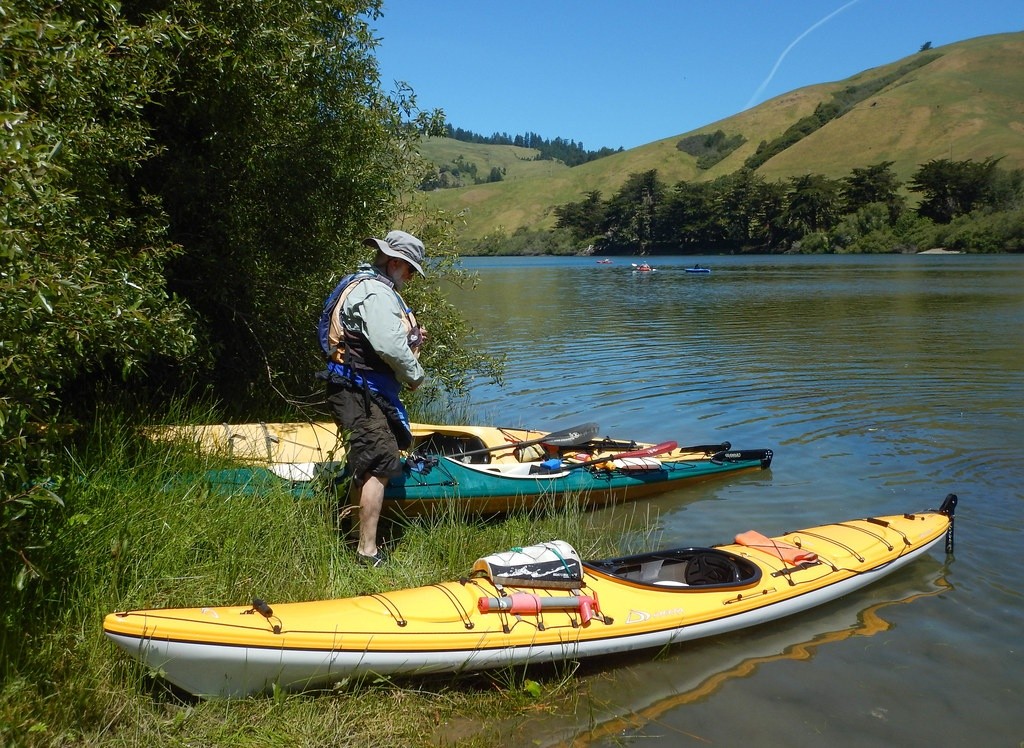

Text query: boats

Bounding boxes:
[425,552,952,748]
[630,263,657,271]
[596,260,613,263]
[686,268,710,273]
[103,490,961,701]
[131,422,775,523]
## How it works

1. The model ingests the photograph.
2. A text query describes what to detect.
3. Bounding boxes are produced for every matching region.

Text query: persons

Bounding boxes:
[604,258,609,262]
[640,261,650,268]
[694,264,701,269]
[319,229,428,571]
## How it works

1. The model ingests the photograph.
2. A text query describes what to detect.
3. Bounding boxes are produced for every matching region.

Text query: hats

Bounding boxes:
[363,231,426,280]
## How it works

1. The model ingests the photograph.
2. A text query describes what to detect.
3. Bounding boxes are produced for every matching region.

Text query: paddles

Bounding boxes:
[538,440,678,476]
[445,422,600,458]
[631,263,642,267]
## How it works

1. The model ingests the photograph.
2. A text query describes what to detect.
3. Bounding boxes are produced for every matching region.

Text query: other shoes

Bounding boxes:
[356,552,385,567]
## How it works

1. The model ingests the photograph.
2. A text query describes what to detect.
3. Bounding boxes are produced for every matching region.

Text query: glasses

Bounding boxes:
[399,258,417,277]
